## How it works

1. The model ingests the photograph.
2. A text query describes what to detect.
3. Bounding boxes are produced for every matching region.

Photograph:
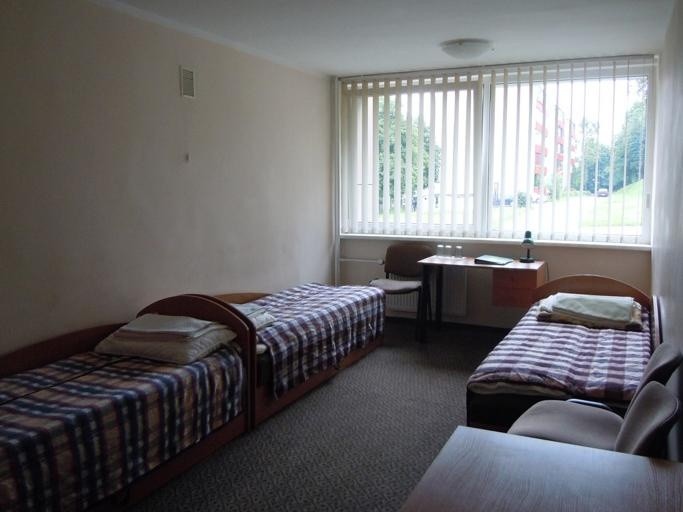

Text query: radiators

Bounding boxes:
[385,266,468,317]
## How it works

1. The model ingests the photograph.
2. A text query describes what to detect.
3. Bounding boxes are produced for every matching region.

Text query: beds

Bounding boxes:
[0,295,252,512]
[184,282,388,427]
[466,274,661,432]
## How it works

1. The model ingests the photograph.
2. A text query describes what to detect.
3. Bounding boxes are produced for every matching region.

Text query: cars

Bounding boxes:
[598,189,608,197]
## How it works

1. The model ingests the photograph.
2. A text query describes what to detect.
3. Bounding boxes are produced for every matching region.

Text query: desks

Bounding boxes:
[397,426,683,512]
[417,255,547,343]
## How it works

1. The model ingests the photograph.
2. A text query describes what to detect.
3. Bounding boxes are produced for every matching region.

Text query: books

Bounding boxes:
[474,253,513,266]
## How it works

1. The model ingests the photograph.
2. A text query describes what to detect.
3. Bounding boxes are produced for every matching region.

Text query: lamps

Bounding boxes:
[442,40,491,58]
[519,231,534,263]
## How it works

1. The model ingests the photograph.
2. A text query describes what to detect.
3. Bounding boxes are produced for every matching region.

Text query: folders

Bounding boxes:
[475,254,514,265]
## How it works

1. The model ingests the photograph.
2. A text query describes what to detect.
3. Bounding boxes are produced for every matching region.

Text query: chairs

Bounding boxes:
[615,381,679,455]
[369,244,435,342]
[507,342,680,449]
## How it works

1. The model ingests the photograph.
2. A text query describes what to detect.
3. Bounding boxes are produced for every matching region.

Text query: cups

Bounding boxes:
[436,245,463,258]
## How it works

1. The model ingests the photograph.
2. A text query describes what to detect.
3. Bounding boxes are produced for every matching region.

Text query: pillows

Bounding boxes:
[537,296,643,332]
[230,303,277,330]
[95,317,238,364]
